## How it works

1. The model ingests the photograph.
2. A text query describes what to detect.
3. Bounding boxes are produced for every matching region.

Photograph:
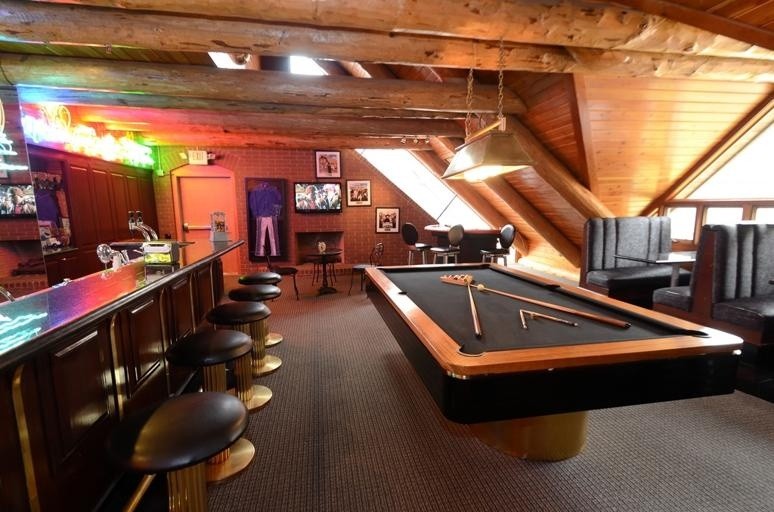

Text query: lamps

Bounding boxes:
[441,36,532,186]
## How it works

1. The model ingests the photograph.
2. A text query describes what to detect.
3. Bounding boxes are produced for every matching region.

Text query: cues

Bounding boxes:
[468,284,483,337]
[519,309,579,329]
[471,283,632,329]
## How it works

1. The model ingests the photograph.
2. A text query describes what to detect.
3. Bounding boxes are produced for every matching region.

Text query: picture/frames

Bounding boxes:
[345,179,372,206]
[316,150,341,178]
[375,206,400,233]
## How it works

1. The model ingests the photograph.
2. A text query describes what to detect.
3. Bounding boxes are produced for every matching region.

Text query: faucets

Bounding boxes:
[136,210,159,241]
[128,210,151,255]
[0,286,15,302]
[97,244,130,265]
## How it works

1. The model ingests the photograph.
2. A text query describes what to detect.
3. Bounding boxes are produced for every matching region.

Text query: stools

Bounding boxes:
[227,284,281,375]
[164,331,255,483]
[102,392,250,512]
[208,301,274,411]
[238,272,283,347]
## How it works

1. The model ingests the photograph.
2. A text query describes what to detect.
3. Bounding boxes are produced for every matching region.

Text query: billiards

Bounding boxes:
[478,285,484,292]
[442,274,473,285]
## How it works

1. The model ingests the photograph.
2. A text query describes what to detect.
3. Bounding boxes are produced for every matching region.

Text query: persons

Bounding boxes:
[0,184,35,214]
[379,213,396,228]
[295,183,341,210]
[350,187,368,200]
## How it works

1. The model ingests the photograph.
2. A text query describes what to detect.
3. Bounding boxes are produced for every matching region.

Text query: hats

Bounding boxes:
[306,185,318,194]
[7,187,23,198]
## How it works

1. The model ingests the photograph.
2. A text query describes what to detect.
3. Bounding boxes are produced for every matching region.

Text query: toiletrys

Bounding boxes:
[113,256,121,269]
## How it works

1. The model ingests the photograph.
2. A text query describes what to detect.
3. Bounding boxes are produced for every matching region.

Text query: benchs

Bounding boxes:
[578,216,691,299]
[653,223,709,321]
[707,223,773,404]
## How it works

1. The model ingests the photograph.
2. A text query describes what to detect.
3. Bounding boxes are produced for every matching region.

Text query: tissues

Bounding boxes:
[141,239,179,265]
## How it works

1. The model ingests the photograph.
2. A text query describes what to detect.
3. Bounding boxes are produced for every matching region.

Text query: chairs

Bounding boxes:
[480,224,516,267]
[430,225,463,264]
[312,241,338,286]
[402,223,431,264]
[347,243,384,298]
[263,245,299,302]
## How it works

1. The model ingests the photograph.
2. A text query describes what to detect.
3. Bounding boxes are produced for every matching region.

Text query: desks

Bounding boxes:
[613,250,698,288]
[308,249,343,295]
[426,224,503,263]
[366,263,743,462]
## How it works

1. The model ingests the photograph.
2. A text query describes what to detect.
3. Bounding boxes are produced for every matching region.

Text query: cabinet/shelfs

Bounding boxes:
[67,151,159,276]
